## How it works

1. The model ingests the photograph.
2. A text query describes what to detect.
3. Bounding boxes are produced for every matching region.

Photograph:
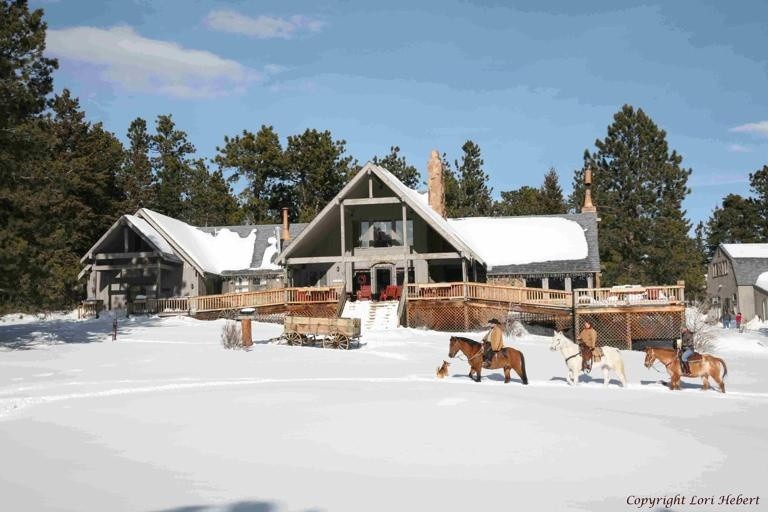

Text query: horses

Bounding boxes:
[644,345,727,393]
[549,329,627,388]
[448,336,527,384]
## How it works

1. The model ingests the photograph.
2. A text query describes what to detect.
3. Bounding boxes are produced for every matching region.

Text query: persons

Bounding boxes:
[577,320,597,354]
[676,323,694,378]
[735,313,741,328]
[482,319,504,367]
[720,308,731,329]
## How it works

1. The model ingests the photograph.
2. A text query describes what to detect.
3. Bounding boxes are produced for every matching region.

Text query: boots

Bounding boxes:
[683,361,692,376]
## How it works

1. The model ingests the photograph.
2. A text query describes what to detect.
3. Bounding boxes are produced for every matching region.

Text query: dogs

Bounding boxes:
[436,359,451,378]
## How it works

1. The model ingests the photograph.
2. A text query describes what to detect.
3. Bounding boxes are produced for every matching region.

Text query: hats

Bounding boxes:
[487,318,501,325]
